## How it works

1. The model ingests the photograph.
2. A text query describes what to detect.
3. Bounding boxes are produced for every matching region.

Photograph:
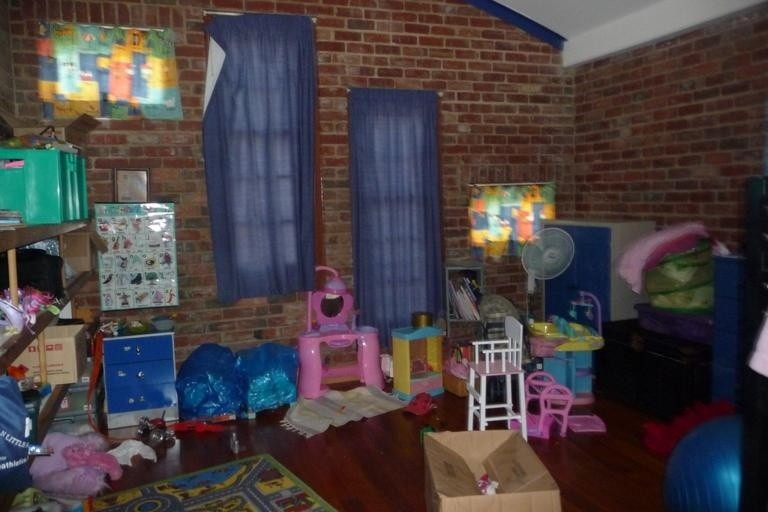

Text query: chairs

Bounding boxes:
[467,314,527,440]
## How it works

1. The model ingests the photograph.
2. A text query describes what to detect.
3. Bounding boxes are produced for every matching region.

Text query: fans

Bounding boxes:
[521,228,573,323]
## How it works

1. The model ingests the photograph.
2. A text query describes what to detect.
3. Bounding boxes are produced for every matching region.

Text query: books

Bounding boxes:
[448,276,481,320]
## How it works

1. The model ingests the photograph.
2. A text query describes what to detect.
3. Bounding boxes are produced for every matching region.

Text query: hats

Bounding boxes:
[404,393,436,416]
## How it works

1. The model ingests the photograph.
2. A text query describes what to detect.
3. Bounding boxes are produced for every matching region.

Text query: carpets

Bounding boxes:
[89,453,339,512]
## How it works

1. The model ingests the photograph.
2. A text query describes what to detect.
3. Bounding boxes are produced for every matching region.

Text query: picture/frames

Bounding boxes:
[114,166,151,202]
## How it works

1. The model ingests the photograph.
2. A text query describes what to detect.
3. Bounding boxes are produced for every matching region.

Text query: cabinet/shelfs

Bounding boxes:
[101,332,179,424]
[0,217,96,512]
[544,217,655,322]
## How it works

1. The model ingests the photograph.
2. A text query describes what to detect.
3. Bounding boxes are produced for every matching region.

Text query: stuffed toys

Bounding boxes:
[62,444,123,482]
[30,432,109,496]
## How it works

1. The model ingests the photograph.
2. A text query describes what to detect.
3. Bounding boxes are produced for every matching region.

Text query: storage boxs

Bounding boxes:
[15,324,88,385]
[423,428,561,512]
